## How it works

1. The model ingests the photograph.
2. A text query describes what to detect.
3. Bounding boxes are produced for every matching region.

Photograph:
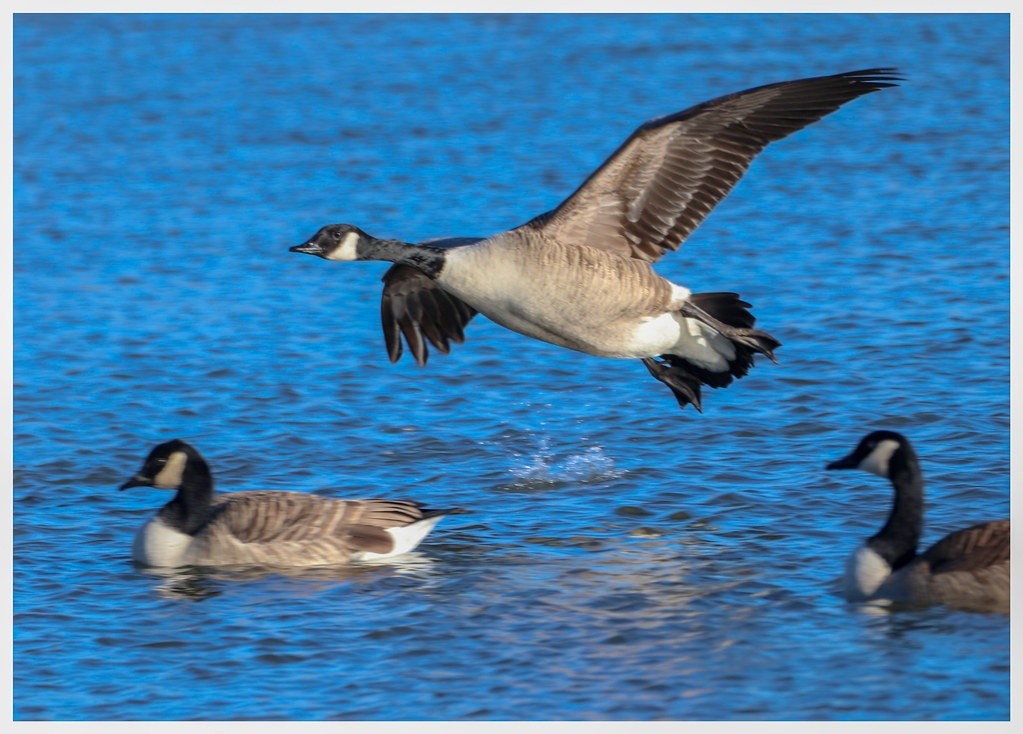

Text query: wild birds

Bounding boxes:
[289,65,907,414]
[824,429,1010,617]
[118,438,479,569]
